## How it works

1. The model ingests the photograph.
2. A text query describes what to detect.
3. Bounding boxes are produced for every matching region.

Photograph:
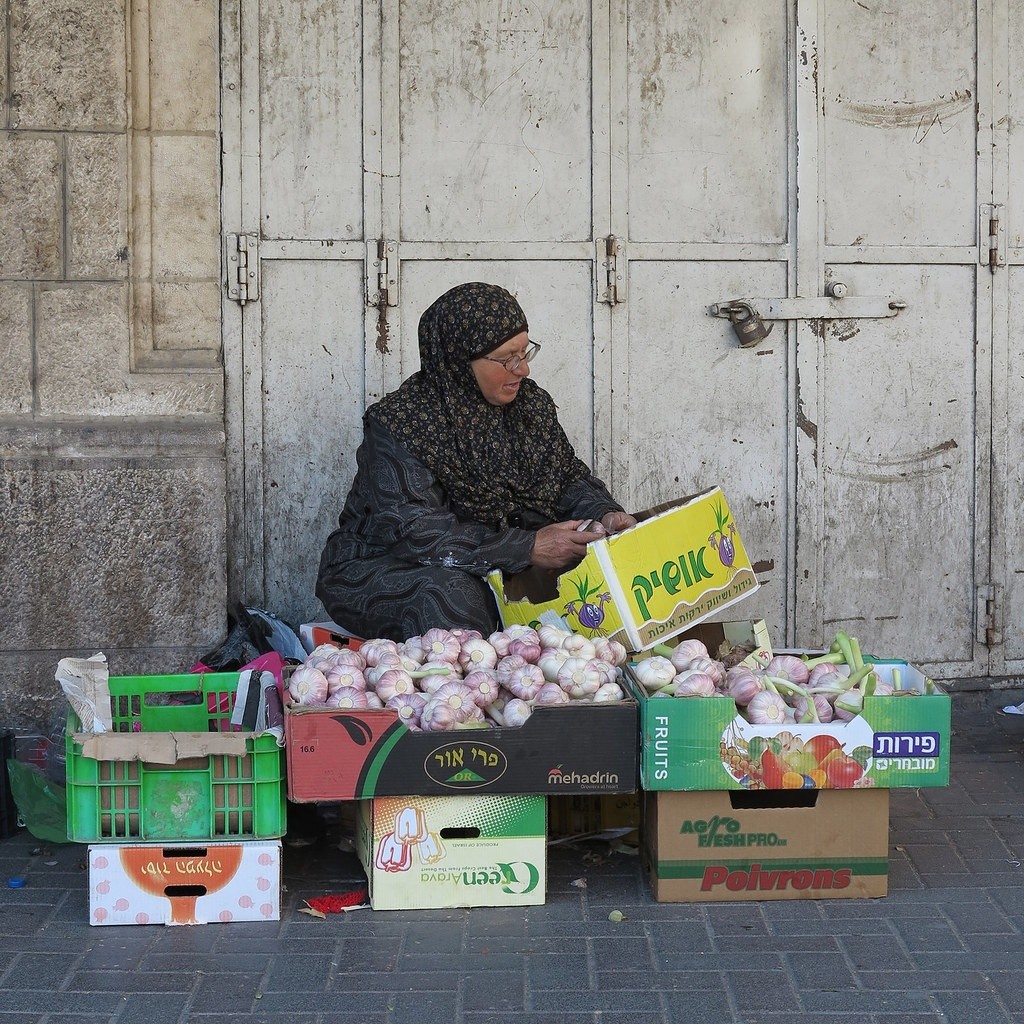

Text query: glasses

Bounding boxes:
[481,340,540,372]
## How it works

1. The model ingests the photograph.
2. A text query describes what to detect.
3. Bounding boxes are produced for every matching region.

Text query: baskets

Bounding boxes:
[65,671,289,842]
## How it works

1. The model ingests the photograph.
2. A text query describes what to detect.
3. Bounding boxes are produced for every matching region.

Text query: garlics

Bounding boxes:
[583,520,607,537]
[637,637,895,727]
[286,625,628,732]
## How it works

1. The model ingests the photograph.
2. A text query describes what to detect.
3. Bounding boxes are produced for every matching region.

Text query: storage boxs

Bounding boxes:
[643,796,891,904]
[632,657,951,791]
[86,842,284,925]
[298,623,362,657]
[357,796,550,912]
[491,484,763,656]
[600,789,645,848]
[282,686,644,795]
[550,793,601,837]
[62,671,288,842]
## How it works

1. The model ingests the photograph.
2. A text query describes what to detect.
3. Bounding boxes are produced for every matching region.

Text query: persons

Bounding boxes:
[315,282,637,643]
